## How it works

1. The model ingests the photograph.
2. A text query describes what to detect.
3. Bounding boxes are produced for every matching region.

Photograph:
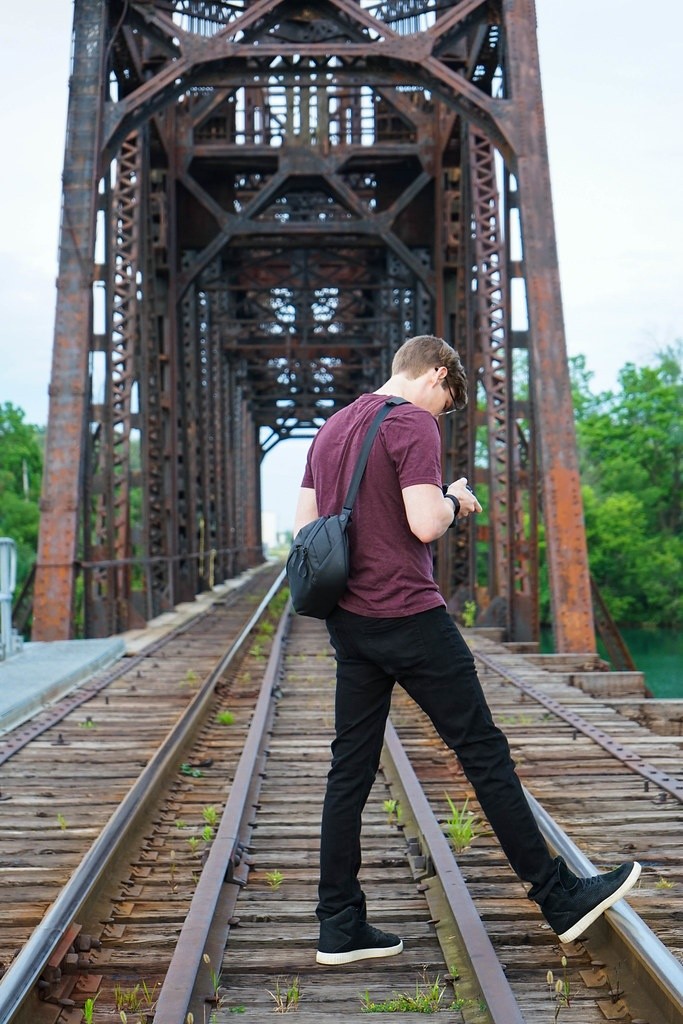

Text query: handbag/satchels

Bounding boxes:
[285,518,351,620]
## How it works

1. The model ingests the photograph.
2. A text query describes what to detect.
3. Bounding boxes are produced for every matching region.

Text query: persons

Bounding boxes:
[297,336,641,965]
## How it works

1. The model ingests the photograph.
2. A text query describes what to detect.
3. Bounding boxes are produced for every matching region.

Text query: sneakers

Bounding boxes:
[527,856,642,945]
[316,902,404,965]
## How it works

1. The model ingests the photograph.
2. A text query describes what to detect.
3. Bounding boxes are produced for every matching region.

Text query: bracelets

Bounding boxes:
[445,494,461,519]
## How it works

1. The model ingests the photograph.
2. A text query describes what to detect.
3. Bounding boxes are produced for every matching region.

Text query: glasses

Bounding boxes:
[434,368,460,416]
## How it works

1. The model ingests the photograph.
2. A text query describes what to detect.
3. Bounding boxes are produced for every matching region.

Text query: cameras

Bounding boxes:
[442,483,474,528]
